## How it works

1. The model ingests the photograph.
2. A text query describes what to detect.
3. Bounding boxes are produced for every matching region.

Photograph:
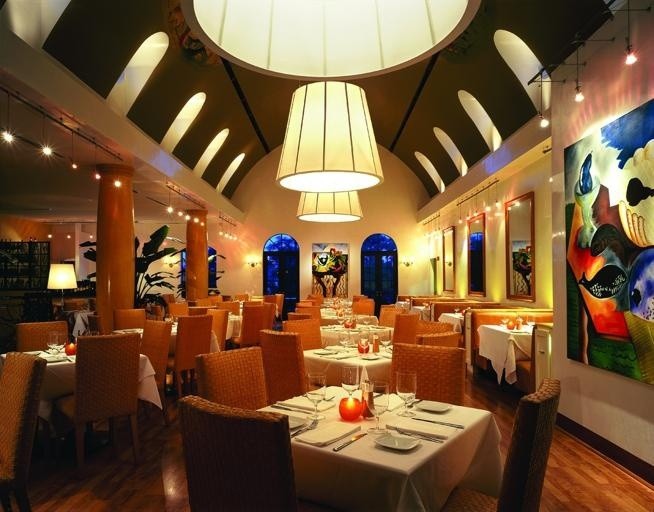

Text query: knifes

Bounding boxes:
[398,428,443,444]
[333,433,367,452]
[413,418,464,429]
[271,405,312,415]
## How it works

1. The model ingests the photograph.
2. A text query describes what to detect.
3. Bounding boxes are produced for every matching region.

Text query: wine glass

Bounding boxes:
[454,305,461,314]
[396,370,418,418]
[501,313,509,329]
[526,314,535,329]
[336,314,343,328]
[338,331,351,351]
[164,314,173,321]
[364,379,390,434]
[363,314,370,328]
[47,330,59,353]
[358,327,370,359]
[307,374,328,420]
[54,333,67,353]
[341,365,361,399]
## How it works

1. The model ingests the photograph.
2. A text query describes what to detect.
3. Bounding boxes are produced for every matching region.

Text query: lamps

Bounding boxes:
[46,263,78,311]
[539,0,637,128]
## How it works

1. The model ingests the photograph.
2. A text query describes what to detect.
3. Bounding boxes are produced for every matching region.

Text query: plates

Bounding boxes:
[362,355,380,361]
[416,400,450,412]
[374,432,421,450]
[313,349,335,355]
[288,416,306,430]
[42,356,67,362]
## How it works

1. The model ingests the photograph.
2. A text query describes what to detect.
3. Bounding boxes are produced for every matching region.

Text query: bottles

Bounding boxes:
[360,379,374,418]
[516,317,524,330]
[372,333,380,354]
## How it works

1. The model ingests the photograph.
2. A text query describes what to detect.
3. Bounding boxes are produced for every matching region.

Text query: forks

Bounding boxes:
[313,393,335,402]
[290,419,319,438]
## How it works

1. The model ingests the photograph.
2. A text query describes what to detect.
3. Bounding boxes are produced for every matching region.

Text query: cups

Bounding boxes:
[379,329,391,351]
